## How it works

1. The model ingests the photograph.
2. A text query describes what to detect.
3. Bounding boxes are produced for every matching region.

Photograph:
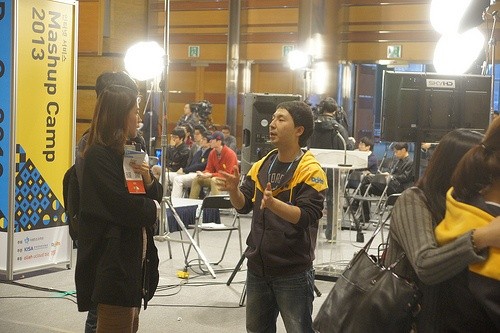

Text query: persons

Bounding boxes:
[176,103,195,126]
[75,71,146,333]
[215,101,329,333]
[346,137,378,189]
[151,126,239,218]
[306,97,350,240]
[381,115,500,333]
[342,143,415,223]
[221,127,237,152]
[75,86,163,333]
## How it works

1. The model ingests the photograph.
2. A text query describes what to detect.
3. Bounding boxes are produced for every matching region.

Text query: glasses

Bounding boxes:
[170,136,179,139]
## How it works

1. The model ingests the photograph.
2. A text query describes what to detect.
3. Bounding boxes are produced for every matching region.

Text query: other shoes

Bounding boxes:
[358,215,370,223]
[327,236,337,243]
[343,203,358,211]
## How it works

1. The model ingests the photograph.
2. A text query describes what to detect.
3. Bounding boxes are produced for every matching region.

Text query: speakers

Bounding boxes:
[241,93,302,174]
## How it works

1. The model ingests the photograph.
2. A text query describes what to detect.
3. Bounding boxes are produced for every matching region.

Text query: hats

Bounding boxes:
[206,131,224,141]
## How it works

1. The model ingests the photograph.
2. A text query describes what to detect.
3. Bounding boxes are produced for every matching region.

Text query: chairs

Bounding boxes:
[203,159,403,253]
[184,194,244,272]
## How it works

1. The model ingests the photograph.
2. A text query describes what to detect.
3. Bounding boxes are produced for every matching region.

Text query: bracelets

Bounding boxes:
[471,229,481,253]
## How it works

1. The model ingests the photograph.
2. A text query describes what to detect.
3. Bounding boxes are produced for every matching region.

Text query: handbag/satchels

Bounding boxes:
[313,213,433,333]
[63,165,80,249]
